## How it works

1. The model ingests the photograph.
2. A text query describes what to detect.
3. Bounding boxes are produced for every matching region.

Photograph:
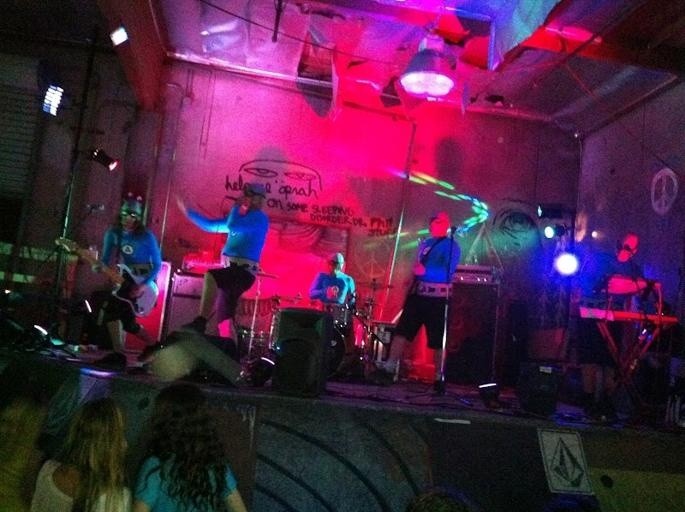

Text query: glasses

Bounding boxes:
[615,245,632,251]
[121,211,140,218]
[243,189,266,200]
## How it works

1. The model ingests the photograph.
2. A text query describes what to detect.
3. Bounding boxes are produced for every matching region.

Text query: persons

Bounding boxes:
[30,397,132,512]
[577,233,645,422]
[0,397,49,511]
[133,384,248,512]
[90,199,163,370]
[310,252,357,312]
[405,487,473,511]
[176,182,270,348]
[375,211,461,397]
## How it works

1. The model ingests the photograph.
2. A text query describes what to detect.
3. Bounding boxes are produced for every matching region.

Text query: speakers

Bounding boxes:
[271,307,334,398]
[443,283,515,394]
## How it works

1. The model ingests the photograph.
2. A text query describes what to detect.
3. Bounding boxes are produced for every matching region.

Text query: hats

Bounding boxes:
[121,199,142,215]
[246,183,266,195]
[331,252,344,264]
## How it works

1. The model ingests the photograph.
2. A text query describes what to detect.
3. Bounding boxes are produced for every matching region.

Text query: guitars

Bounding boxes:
[55,236,160,318]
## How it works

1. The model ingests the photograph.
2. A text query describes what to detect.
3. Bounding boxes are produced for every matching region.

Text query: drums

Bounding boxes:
[321,329,356,380]
[371,322,397,344]
[322,303,353,329]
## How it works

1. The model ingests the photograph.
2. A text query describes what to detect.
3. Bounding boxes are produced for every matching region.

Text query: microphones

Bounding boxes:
[87,202,105,211]
[449,224,457,238]
[226,194,239,202]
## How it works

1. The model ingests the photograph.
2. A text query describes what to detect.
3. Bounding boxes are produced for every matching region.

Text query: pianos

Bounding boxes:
[579,302,678,325]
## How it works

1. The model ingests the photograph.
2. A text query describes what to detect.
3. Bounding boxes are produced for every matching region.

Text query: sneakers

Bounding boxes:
[434,379,444,390]
[138,340,163,364]
[182,319,206,332]
[91,353,126,369]
[373,358,399,375]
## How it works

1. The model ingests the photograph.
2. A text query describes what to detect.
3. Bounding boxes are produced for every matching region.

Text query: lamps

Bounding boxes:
[538,202,571,219]
[544,223,566,239]
[92,147,118,172]
[399,48,456,96]
